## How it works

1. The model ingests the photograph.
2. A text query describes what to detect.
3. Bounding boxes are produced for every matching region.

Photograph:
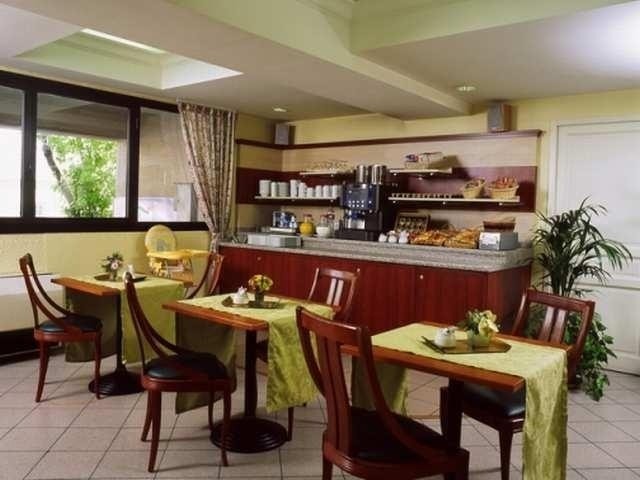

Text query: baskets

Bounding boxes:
[404,155,459,169]
[410,223,484,249]
[484,180,519,200]
[459,177,488,199]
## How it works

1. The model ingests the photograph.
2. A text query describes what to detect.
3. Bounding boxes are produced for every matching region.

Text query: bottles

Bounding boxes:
[315,214,331,237]
[299,214,315,237]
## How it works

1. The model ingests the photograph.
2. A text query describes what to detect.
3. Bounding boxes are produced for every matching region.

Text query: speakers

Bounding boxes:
[487,104,511,132]
[273,123,290,146]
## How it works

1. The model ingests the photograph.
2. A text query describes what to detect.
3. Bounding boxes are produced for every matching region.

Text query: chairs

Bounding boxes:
[144,225,194,287]
